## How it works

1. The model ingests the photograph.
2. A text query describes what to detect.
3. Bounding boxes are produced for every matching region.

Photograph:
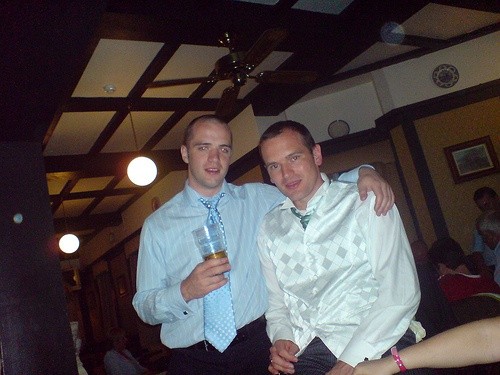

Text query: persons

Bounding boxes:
[410,240,457,340]
[427,236,500,300]
[253,120,427,375]
[132,115,395,375]
[104,326,151,375]
[352,317,500,375]
[472,186,500,287]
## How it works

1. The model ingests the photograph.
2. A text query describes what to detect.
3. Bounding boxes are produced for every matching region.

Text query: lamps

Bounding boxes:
[59,195,79,254]
[127,104,158,186]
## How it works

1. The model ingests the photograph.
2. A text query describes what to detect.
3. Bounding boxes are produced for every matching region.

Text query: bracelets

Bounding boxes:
[391,346,406,372]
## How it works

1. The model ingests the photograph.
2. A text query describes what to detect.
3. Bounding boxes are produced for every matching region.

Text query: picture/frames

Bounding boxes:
[442,136,500,183]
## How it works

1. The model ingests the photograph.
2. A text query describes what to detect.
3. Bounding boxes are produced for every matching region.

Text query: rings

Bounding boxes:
[270,359,273,362]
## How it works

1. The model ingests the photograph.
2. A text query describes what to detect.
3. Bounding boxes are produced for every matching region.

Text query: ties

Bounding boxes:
[290,206,313,230]
[199,193,237,354]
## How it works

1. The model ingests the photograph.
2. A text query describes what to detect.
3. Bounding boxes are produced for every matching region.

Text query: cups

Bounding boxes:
[191,223,231,275]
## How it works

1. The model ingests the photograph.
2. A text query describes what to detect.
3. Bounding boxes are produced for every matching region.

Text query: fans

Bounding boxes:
[146,24,318,118]
